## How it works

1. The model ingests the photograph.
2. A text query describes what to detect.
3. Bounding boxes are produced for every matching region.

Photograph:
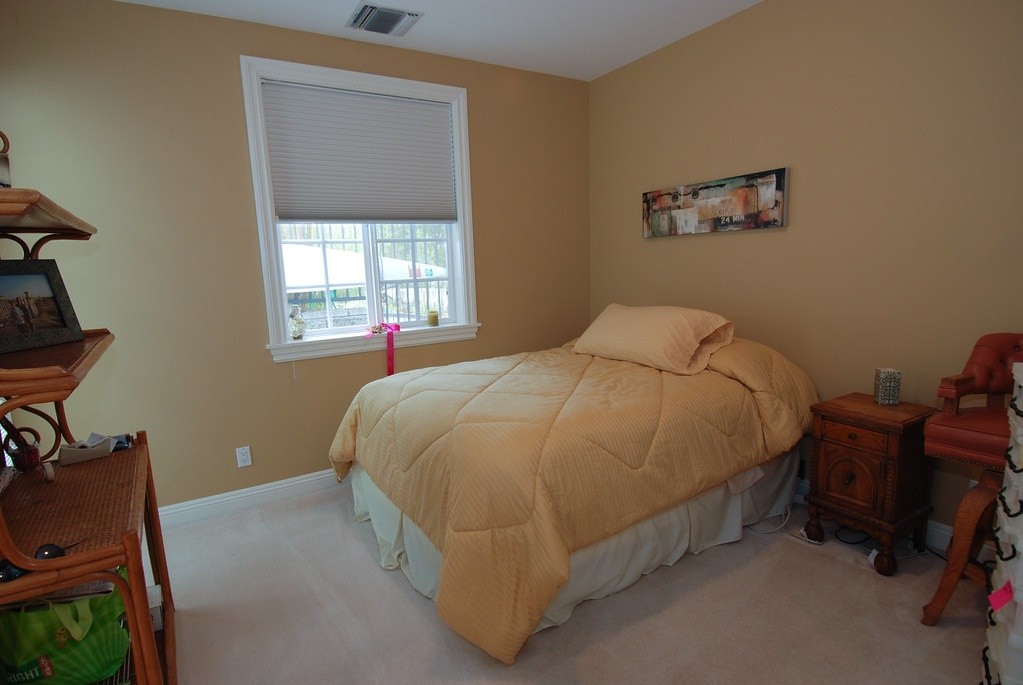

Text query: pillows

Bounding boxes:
[573,302,735,376]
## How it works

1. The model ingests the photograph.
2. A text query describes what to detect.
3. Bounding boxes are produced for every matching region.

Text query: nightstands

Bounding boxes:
[802,392,940,578]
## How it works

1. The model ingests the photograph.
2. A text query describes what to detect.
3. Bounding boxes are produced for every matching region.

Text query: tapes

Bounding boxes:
[42,462,55,482]
[75,438,109,449]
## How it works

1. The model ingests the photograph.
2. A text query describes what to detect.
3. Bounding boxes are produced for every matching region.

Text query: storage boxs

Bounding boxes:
[979,363,1023,685]
[873,368,901,404]
[146,585,163,632]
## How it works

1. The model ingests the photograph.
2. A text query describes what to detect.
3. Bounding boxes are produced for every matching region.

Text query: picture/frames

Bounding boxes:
[0,259,85,355]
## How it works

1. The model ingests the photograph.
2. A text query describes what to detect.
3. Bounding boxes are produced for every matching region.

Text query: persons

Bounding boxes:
[9,298,35,334]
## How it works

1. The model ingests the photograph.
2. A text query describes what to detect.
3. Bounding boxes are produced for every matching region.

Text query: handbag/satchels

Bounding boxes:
[0,566,132,685]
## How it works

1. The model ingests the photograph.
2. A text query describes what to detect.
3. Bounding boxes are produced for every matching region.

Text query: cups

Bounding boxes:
[8,440,40,473]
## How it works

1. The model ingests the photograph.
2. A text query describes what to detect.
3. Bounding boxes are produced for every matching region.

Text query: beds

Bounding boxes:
[329,336,820,665]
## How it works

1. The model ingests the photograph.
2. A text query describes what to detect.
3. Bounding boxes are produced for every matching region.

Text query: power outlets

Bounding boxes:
[236,445,252,468]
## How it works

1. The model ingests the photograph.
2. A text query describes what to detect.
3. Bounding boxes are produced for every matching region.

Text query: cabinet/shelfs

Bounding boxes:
[0,188,177,685]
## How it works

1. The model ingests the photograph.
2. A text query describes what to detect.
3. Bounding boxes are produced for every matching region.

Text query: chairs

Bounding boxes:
[919,332,1023,627]
